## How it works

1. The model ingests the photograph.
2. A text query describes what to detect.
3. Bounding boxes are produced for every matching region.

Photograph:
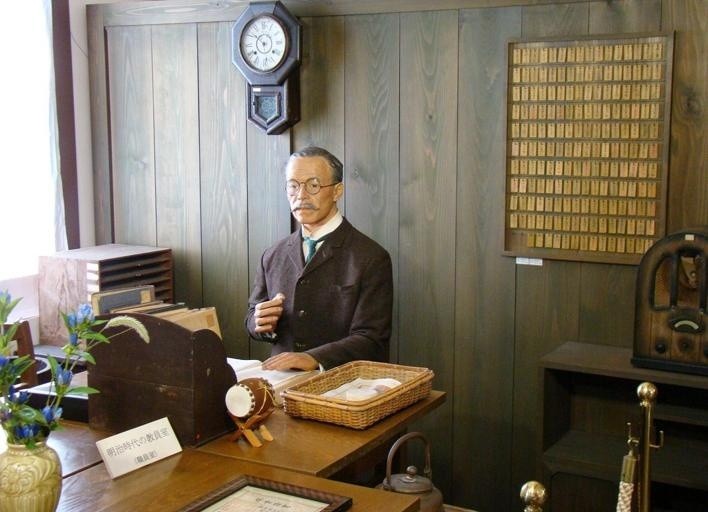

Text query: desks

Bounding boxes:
[51,444,423,512]
[0,407,118,482]
[0,340,451,485]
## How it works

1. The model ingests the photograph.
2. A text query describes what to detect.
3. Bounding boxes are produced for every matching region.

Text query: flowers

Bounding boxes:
[0,282,159,451]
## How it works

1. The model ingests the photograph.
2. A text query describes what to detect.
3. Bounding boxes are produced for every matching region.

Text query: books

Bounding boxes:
[221,354,322,408]
[90,282,223,347]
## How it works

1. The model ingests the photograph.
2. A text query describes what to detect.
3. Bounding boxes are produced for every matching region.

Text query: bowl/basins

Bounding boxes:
[1,355,55,382]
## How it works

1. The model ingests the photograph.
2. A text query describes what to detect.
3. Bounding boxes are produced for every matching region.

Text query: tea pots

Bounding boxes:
[373,432,444,512]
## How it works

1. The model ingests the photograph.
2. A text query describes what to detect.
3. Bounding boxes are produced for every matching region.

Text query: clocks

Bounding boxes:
[228,1,310,140]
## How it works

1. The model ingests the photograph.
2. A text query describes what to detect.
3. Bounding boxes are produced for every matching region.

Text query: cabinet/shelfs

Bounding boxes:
[32,238,178,350]
[526,332,708,512]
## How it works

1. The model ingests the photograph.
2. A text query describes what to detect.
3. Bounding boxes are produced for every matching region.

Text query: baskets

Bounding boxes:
[279,358,436,432]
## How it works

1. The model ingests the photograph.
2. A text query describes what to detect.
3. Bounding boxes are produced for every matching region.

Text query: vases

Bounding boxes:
[0,435,66,512]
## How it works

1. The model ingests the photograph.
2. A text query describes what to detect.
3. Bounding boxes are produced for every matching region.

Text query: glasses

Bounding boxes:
[284,175,340,196]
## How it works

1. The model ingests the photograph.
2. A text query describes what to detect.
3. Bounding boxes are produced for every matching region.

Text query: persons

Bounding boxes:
[239,145,392,373]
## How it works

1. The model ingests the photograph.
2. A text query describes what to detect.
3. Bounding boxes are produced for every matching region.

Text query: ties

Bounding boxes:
[303,233,332,267]
